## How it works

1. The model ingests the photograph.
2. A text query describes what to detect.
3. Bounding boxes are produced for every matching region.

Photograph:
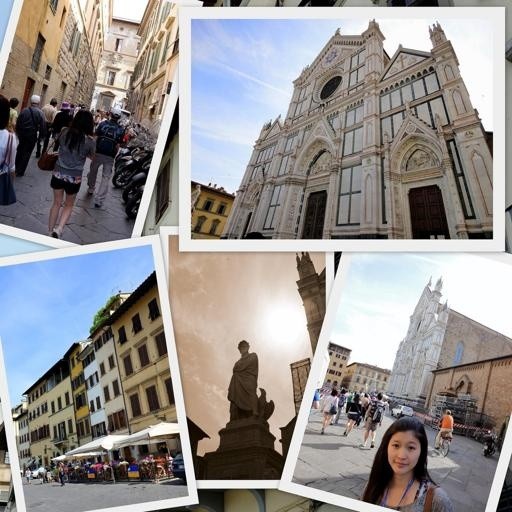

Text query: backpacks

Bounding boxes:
[96,120,119,155]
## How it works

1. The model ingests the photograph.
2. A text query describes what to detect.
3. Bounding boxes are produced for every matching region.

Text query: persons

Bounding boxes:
[40,453,175,487]
[25,467,31,483]
[361,417,454,512]
[0,95,126,238]
[312,389,389,448]
[434,409,454,449]
[227,340,259,419]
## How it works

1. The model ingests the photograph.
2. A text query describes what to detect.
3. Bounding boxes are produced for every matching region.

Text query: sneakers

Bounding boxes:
[52,229,64,241]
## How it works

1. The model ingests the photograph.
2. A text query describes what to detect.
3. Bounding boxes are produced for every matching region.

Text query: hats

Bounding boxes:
[31,94,41,104]
[59,101,72,111]
[112,105,122,117]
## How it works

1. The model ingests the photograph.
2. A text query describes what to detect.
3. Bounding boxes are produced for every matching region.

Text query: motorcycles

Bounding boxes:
[482,431,499,457]
[111,107,162,220]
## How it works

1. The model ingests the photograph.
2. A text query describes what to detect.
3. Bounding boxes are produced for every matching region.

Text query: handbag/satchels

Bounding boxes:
[38,128,68,171]
[1,134,16,206]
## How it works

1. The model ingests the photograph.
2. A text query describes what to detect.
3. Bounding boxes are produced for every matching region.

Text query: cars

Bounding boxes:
[468,428,487,443]
[30,465,49,479]
[171,452,186,482]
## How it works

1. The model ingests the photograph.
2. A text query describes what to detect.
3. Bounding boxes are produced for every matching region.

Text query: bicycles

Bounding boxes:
[432,427,454,457]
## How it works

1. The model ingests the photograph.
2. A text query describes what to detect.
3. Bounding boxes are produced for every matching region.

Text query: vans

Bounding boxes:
[391,404,414,419]
